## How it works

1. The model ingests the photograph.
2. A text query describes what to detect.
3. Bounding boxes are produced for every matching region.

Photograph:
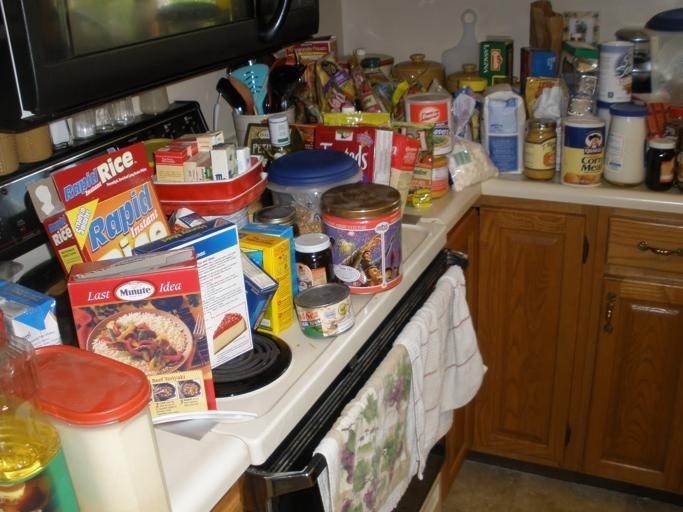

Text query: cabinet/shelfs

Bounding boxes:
[471,193,600,486]
[581,203,682,502]
[437,206,471,501]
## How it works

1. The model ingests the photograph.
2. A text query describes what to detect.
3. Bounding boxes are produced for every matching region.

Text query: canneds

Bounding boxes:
[524,118,557,180]
[431,156,449,199]
[646,138,676,190]
[252,204,300,238]
[294,282,354,339]
[294,231,335,293]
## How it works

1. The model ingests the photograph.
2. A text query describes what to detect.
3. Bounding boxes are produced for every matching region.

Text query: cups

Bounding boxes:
[231,105,296,149]
[0,124,53,176]
[70,85,169,141]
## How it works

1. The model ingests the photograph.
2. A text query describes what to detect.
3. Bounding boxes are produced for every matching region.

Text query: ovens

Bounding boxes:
[242,243,470,511]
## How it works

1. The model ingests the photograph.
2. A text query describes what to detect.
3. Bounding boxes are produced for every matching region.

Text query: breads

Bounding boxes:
[213,312,247,354]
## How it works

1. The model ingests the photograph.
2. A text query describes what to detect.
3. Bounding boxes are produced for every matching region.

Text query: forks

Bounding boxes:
[184,314,205,369]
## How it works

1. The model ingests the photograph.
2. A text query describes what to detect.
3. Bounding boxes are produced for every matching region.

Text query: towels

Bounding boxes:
[388,256,492,482]
[309,341,417,511]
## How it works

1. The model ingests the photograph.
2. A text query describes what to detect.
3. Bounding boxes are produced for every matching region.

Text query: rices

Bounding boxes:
[92,311,188,377]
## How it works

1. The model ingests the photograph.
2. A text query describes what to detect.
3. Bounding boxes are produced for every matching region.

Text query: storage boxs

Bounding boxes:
[638,5,683,116]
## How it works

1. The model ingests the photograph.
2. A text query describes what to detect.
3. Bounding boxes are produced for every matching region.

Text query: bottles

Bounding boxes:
[0,309,79,511]
[353,48,483,103]
[268,114,291,147]
[600,103,647,188]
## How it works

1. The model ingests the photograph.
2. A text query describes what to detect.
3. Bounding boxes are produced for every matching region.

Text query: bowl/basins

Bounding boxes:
[179,381,200,397]
[155,383,176,400]
[264,149,363,236]
[85,308,192,378]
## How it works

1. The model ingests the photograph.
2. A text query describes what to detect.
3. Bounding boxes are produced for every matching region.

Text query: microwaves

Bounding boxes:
[0,1,320,136]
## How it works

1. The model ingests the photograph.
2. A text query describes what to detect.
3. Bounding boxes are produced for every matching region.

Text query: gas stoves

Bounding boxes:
[0,221,447,455]
